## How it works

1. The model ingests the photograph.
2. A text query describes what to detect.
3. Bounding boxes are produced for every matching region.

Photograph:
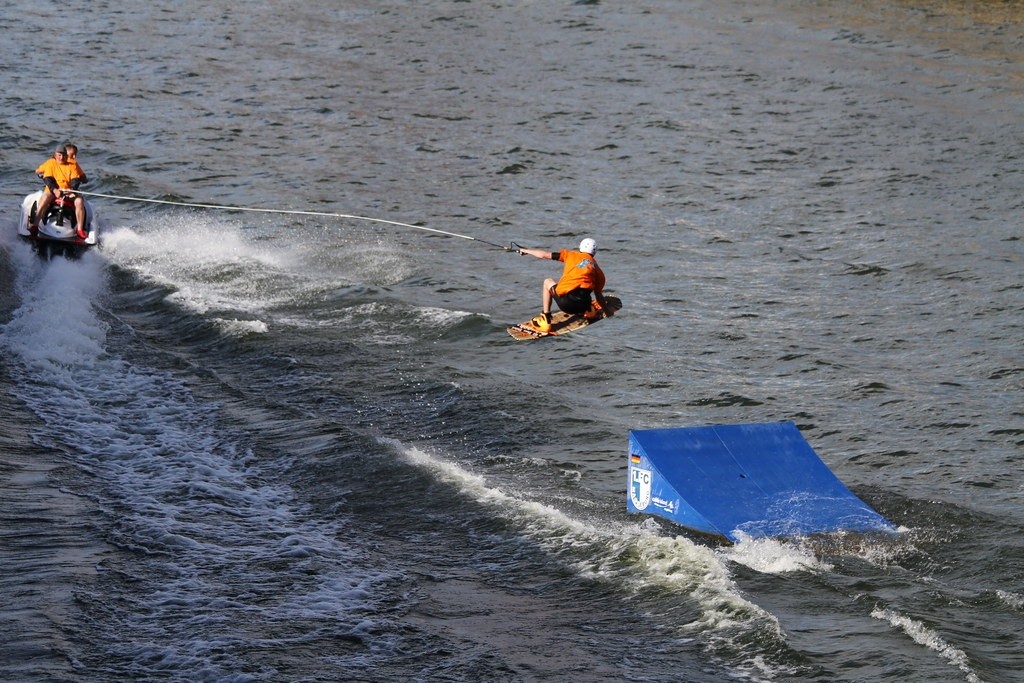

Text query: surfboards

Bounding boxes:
[506,295,623,341]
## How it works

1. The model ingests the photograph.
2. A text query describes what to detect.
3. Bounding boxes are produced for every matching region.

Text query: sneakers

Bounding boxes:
[584,305,596,318]
[531,312,551,333]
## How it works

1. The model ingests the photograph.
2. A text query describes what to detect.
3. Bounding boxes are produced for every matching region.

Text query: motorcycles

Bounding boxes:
[18,189,99,258]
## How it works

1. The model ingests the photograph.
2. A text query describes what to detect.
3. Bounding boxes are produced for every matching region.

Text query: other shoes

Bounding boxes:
[77,230,87,239]
[30,225,38,235]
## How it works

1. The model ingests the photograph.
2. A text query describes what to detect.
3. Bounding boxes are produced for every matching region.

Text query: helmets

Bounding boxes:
[580,238,598,257]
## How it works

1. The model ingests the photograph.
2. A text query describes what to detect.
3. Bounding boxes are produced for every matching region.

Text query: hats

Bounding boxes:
[56,145,67,154]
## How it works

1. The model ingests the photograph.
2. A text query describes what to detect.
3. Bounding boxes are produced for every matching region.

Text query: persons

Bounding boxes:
[29,143,88,238]
[520,238,606,333]
